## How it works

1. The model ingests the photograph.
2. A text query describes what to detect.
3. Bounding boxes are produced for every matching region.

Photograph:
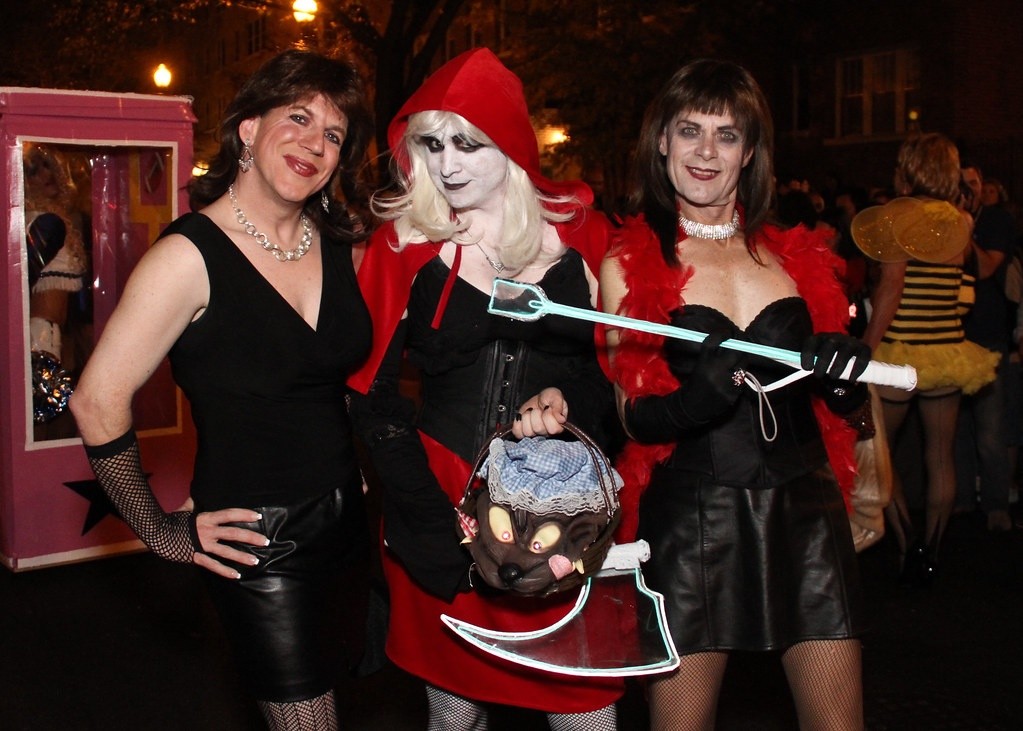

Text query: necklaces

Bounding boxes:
[456,213,507,274]
[228,183,315,261]
[678,209,743,247]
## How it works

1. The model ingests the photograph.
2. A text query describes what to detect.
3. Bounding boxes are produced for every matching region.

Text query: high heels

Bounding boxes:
[898,540,930,589]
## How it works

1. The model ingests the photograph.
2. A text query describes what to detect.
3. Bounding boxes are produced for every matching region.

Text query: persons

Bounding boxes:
[364,44,683,731]
[591,62,871,729]
[75,50,376,729]
[24,144,90,364]
[745,130,1023,583]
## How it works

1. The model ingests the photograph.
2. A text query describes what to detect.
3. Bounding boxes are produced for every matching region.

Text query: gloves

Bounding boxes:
[623,331,749,446]
[801,334,871,416]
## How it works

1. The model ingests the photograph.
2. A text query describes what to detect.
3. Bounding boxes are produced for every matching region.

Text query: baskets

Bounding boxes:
[455,415,618,595]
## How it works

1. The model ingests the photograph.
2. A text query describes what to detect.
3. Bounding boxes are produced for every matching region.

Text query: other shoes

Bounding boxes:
[987,507,1012,532]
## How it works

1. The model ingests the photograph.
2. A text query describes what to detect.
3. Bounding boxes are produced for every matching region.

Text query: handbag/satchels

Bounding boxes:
[999,255,1023,305]
